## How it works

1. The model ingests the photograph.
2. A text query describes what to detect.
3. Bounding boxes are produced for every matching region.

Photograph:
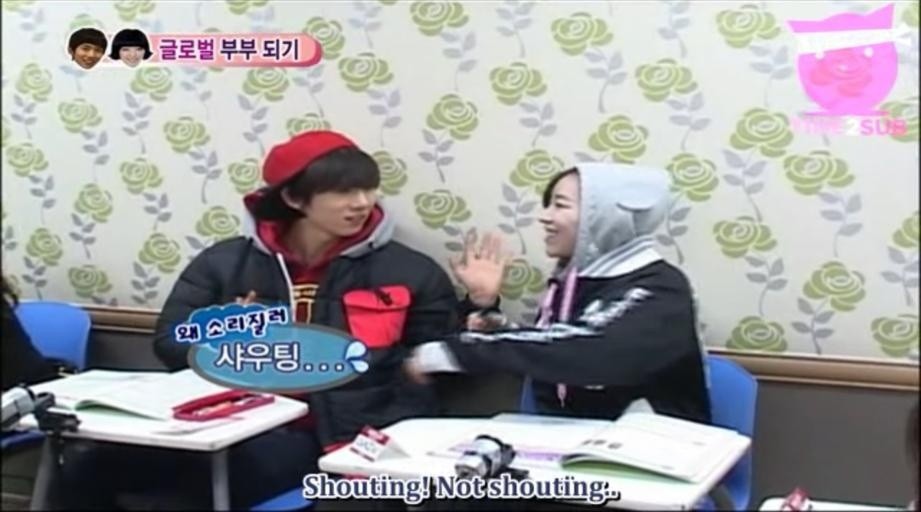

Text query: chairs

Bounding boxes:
[517,353,758,512]
[0,299,93,511]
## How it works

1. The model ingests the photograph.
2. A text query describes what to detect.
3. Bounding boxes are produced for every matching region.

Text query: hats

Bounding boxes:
[263,130,357,186]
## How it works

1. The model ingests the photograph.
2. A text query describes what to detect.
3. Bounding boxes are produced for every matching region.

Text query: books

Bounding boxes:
[430,404,742,486]
[30,368,229,423]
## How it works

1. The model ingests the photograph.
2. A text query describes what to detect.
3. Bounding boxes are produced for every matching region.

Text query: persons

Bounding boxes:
[402,160,716,512]
[107,28,153,65]
[67,26,106,68]
[43,129,470,511]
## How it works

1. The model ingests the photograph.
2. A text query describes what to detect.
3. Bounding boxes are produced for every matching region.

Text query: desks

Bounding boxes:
[317,413,754,512]
[2,369,310,512]
